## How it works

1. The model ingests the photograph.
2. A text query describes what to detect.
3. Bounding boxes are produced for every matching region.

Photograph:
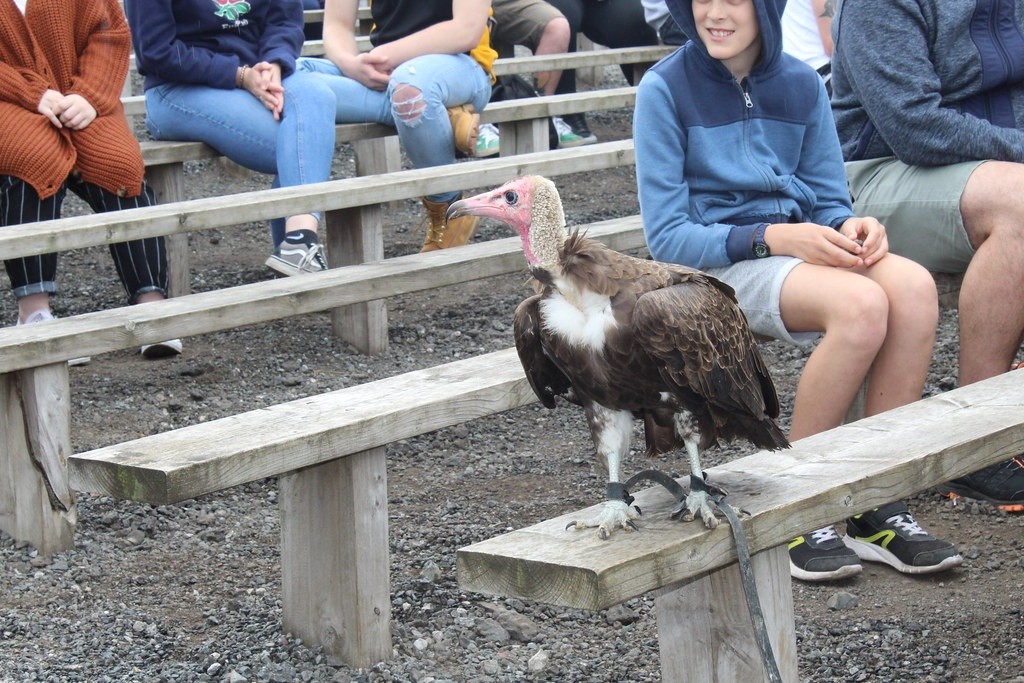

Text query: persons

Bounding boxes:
[633,0,963,580]
[828,0,1023,515]
[0,0,184,365]
[545,0,837,143]
[124,0,335,278]
[477,0,598,158]
[297,0,494,253]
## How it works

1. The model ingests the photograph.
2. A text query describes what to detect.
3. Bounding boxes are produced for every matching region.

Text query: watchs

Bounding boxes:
[751,222,771,258]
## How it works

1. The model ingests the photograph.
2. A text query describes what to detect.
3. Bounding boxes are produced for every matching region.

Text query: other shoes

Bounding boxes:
[140,336,183,359]
[15,310,92,366]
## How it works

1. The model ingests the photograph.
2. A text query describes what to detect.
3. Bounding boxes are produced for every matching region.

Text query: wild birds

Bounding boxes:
[443,173,792,542]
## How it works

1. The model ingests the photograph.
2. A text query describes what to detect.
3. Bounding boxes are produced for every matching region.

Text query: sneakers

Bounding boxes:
[551,116,598,148]
[446,102,479,154]
[474,122,502,157]
[786,523,863,580]
[263,239,331,278]
[937,454,1024,510]
[418,191,478,254]
[842,501,964,574]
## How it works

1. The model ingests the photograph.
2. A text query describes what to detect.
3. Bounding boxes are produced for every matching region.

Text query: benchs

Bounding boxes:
[0,6,1024,683]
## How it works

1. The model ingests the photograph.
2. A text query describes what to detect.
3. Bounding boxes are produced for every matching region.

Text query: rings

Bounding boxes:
[259,96,264,102]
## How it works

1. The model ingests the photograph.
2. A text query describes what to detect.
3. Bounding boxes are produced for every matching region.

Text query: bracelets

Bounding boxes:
[240,64,248,88]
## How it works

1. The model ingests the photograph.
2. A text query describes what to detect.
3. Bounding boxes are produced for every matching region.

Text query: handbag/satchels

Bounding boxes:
[489,73,559,150]
